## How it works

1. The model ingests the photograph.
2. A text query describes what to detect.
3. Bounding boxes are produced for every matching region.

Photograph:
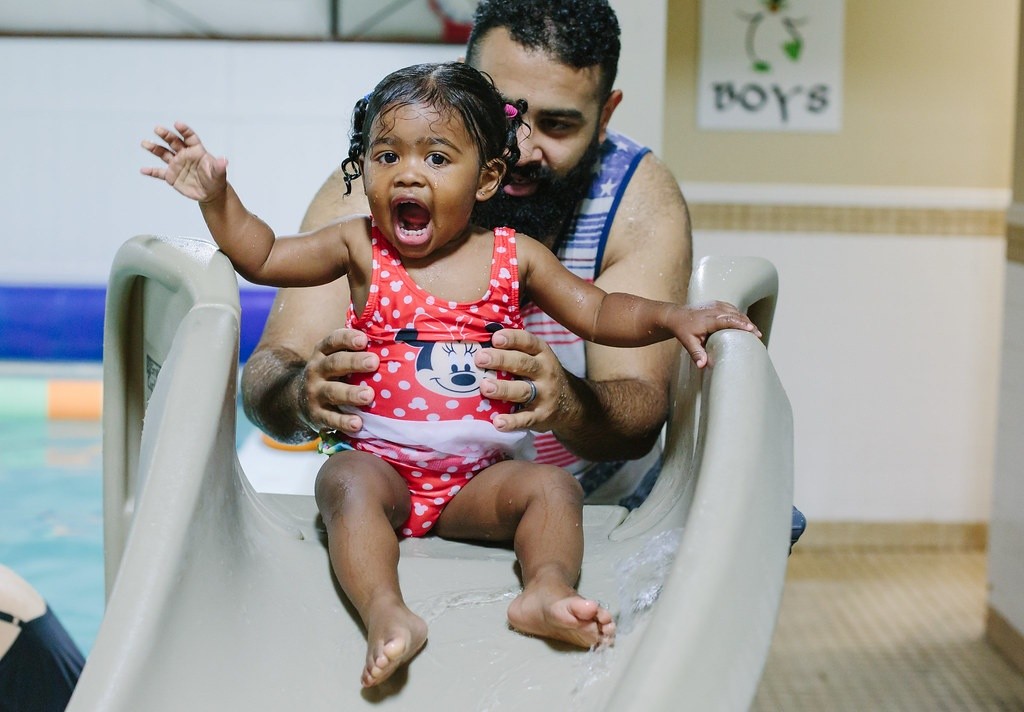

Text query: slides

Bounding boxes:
[63,233,797,712]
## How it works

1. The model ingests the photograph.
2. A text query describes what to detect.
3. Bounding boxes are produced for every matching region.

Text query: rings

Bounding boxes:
[522,379,538,406]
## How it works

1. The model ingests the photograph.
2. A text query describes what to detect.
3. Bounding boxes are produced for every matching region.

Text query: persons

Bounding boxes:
[139,61,762,689]
[241,0,694,509]
[0,563,86,712]
